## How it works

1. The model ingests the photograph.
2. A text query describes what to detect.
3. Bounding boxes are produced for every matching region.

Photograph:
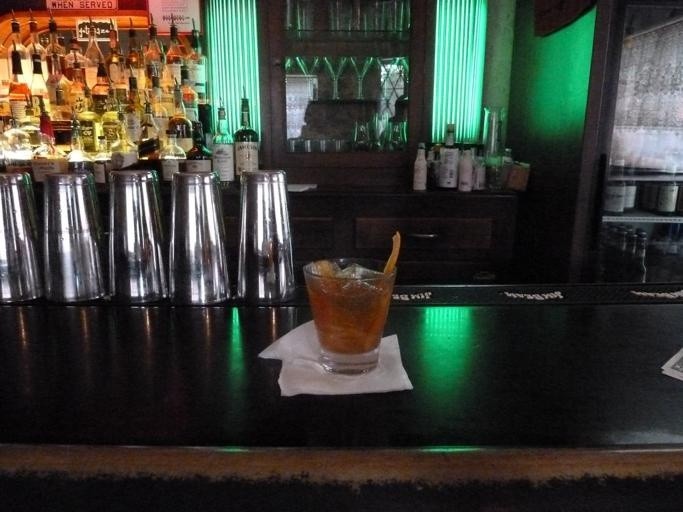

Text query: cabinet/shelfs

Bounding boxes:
[600,172,682,282]
[266,1,424,163]
[1,19,205,168]
[221,187,517,286]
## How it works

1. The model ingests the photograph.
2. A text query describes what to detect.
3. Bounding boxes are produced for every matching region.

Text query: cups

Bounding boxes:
[288,111,406,151]
[236,169,297,305]
[0,171,41,305]
[108,170,169,305]
[302,258,398,375]
[41,170,105,304]
[168,170,231,307]
[506,161,531,192]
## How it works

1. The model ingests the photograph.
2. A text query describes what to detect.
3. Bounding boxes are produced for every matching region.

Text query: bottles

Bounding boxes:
[0,23,259,192]
[594,152,680,283]
[411,124,513,193]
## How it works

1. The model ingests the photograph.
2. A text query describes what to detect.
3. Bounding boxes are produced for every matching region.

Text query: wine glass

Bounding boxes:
[284,55,409,101]
